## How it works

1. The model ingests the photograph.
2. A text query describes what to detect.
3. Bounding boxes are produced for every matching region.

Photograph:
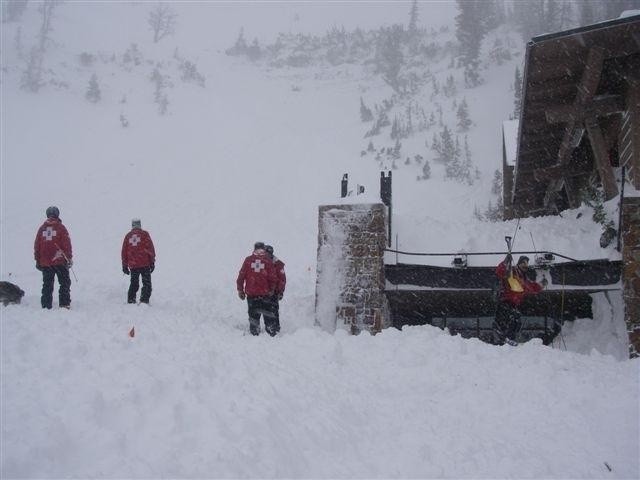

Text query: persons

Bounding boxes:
[263,245,286,334]
[121,217,156,303]
[236,242,277,337]
[492,254,549,346]
[34,206,73,310]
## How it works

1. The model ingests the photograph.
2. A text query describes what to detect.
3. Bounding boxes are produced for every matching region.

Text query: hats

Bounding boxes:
[518,254,529,265]
[252,241,274,253]
[131,218,143,228]
[46,206,59,217]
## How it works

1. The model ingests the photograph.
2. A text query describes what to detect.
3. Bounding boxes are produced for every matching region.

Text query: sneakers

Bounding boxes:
[494,339,517,346]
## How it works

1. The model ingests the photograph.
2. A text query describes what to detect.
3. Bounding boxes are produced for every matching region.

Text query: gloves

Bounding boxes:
[503,254,512,266]
[236,290,285,302]
[540,278,549,287]
[121,264,155,276]
[35,260,74,271]
